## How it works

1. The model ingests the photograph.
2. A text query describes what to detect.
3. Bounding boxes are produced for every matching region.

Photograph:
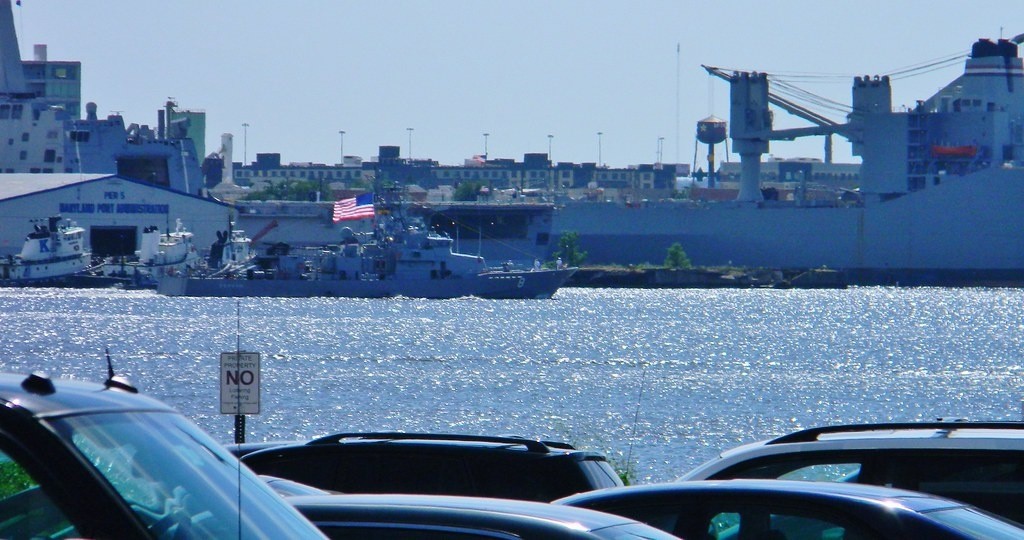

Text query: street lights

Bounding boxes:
[407,128,414,165]
[483,133,490,167]
[547,135,554,168]
[339,131,345,163]
[659,137,665,170]
[242,123,249,167]
[597,132,603,168]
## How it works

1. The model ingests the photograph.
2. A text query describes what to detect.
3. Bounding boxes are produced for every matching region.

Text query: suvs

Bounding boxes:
[239,429,626,504]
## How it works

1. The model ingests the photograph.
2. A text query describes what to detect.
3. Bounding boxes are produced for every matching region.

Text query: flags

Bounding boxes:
[328,190,375,222]
[473,154,486,163]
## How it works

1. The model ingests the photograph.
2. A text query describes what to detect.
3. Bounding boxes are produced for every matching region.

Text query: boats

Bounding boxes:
[0,214,92,287]
[472,265,579,300]
[218,221,261,279]
[103,218,201,288]
[527,36,1024,289]
[155,182,501,299]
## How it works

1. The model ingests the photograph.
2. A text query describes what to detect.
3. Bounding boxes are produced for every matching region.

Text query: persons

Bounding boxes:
[534,257,541,271]
[555,257,562,269]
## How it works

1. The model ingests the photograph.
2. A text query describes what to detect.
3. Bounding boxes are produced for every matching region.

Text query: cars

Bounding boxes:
[550,479,1024,540]
[283,493,684,540]
[0,370,333,540]
[222,442,305,460]
[674,421,1024,528]
